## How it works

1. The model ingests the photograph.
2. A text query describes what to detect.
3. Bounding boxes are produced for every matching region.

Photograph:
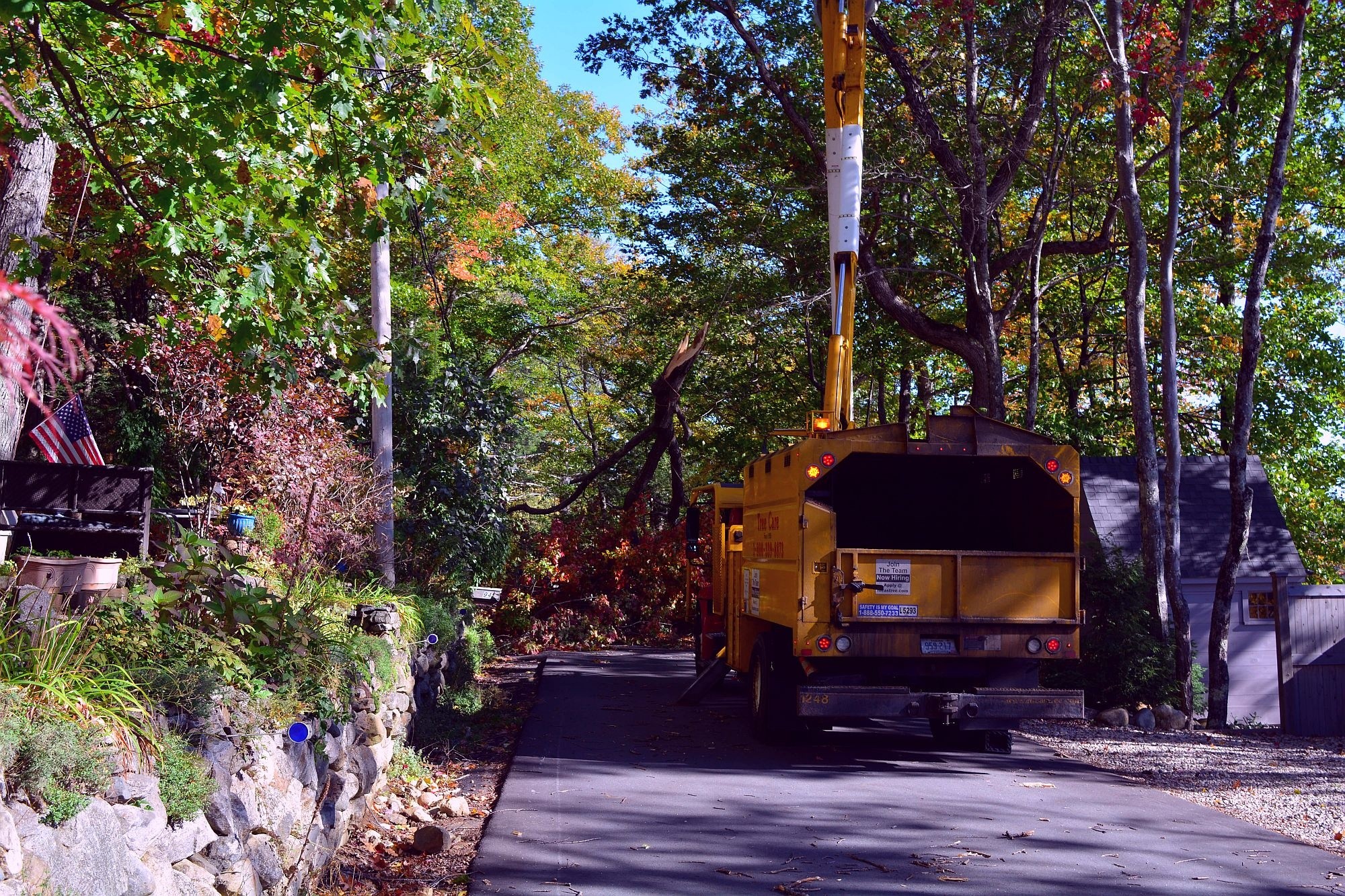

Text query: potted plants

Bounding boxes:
[19,545,89,594]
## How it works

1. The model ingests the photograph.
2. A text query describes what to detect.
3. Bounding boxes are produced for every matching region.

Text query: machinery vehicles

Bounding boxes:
[666,0,1085,753]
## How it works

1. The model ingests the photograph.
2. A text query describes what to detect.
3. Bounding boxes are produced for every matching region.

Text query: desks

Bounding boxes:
[152,508,221,564]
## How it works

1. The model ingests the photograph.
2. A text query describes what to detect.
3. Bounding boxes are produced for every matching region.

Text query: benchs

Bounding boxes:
[0,459,155,562]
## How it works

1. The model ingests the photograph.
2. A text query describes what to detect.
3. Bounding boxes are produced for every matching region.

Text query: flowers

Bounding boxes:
[234,503,249,515]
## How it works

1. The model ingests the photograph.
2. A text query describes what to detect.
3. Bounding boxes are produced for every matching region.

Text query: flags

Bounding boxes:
[30,393,105,466]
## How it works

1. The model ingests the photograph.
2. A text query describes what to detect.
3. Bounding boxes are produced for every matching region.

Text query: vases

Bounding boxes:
[228,514,255,536]
[81,557,124,589]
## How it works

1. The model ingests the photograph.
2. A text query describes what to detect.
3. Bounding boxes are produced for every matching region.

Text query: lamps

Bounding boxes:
[226,720,312,743]
[457,608,468,615]
[406,633,440,648]
